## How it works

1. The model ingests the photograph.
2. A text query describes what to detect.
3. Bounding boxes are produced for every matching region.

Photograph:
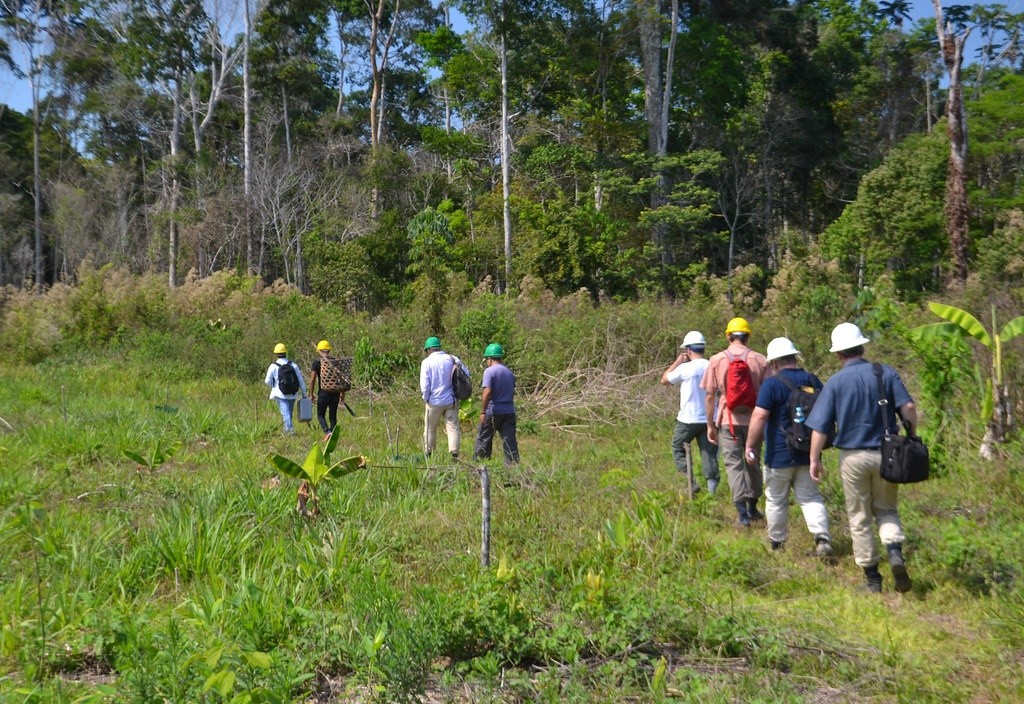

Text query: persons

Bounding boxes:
[310,340,345,443]
[743,336,836,564]
[420,336,471,462]
[264,342,307,434]
[473,343,521,464]
[659,331,719,500]
[803,323,917,592]
[698,317,770,527]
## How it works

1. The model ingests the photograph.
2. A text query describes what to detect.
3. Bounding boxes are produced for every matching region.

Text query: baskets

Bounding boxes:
[319,357,353,393]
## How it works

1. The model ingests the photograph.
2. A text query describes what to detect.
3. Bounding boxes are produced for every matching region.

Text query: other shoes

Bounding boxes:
[815,538,832,557]
[887,550,912,592]
[746,509,764,523]
[321,429,332,443]
[683,484,700,492]
[768,542,786,556]
[738,513,750,526]
[855,578,882,593]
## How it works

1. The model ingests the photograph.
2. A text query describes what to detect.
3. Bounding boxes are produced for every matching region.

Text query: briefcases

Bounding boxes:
[297,396,312,422]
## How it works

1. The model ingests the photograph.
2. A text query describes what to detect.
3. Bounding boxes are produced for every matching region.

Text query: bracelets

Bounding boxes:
[481,412,486,414]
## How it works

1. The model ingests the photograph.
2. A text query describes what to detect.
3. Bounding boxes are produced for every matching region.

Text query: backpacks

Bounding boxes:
[272,360,300,394]
[717,349,759,413]
[773,374,834,451]
[450,354,472,401]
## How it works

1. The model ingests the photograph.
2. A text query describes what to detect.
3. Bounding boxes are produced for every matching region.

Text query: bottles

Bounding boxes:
[793,408,804,422]
[682,353,690,362]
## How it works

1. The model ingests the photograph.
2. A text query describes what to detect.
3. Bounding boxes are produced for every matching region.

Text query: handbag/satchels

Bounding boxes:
[879,432,929,484]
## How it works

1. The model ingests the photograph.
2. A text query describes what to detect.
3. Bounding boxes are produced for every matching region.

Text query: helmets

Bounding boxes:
[766,337,800,362]
[828,322,870,353]
[482,344,504,359]
[678,330,706,349]
[725,318,751,338]
[315,339,331,352]
[273,343,287,353]
[423,337,441,351]
